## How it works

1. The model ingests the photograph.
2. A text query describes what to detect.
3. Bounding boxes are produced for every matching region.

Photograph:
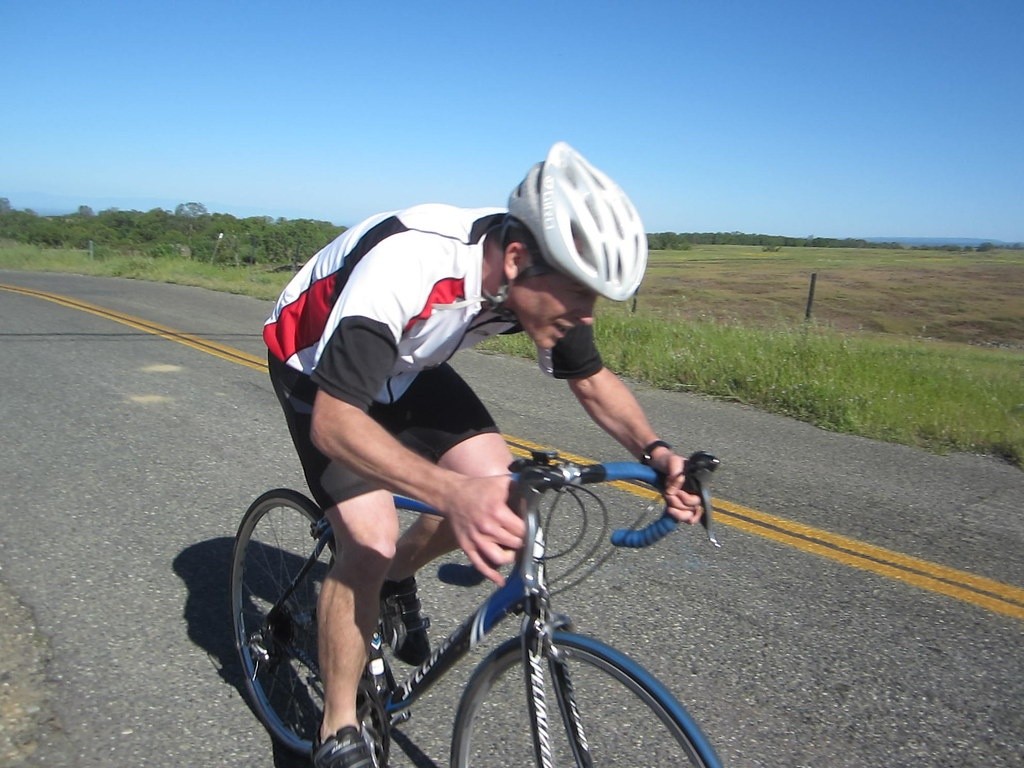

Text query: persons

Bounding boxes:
[262,141,701,768]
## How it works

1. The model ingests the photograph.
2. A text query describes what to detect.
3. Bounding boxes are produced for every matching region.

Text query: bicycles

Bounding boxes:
[229,447,729,768]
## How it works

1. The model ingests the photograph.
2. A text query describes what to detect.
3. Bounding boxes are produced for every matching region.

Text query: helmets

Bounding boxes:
[507,140,647,300]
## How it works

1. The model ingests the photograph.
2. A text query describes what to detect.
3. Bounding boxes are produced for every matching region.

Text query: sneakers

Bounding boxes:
[311,722,374,768]
[375,573,432,666]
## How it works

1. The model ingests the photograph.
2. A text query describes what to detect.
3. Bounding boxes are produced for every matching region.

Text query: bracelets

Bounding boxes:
[641,441,672,464]
